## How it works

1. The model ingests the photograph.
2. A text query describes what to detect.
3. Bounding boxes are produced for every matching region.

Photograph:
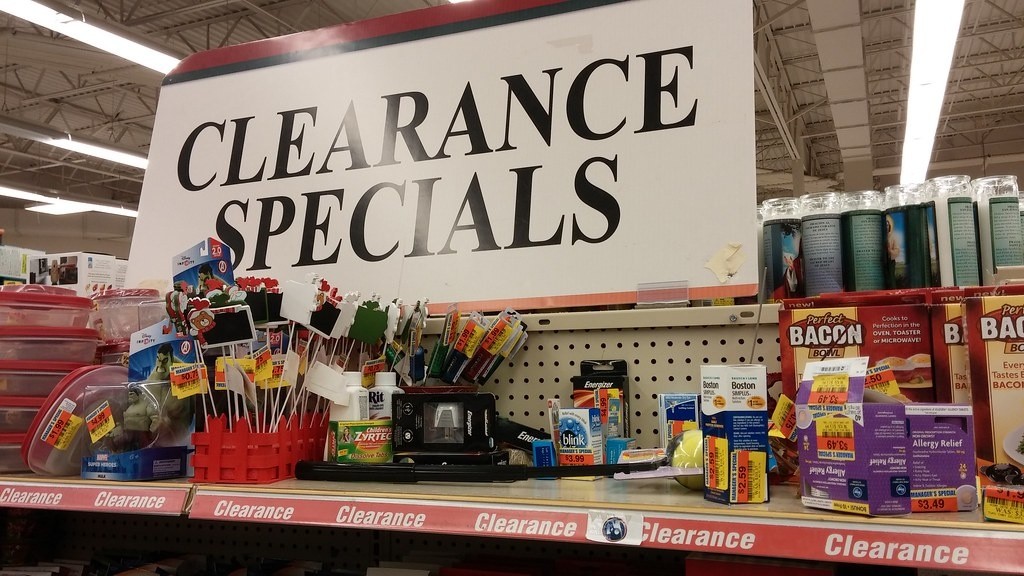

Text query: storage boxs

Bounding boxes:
[81,380,190,483]
[700,363,768,505]
[559,407,603,466]
[657,394,701,449]
[778,284,1024,518]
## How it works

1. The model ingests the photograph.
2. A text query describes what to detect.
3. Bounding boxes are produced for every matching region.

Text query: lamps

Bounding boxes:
[0,115,148,169]
[0,182,139,218]
[0,1,187,75]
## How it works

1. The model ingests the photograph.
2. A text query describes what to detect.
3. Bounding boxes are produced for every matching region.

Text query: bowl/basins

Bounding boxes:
[0,285,128,477]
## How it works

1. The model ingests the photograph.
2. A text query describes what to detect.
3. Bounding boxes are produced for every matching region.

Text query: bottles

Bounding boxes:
[329,371,404,422]
[89,289,168,344]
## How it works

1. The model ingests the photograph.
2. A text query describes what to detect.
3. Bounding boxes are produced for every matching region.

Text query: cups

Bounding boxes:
[760,174,1024,303]
